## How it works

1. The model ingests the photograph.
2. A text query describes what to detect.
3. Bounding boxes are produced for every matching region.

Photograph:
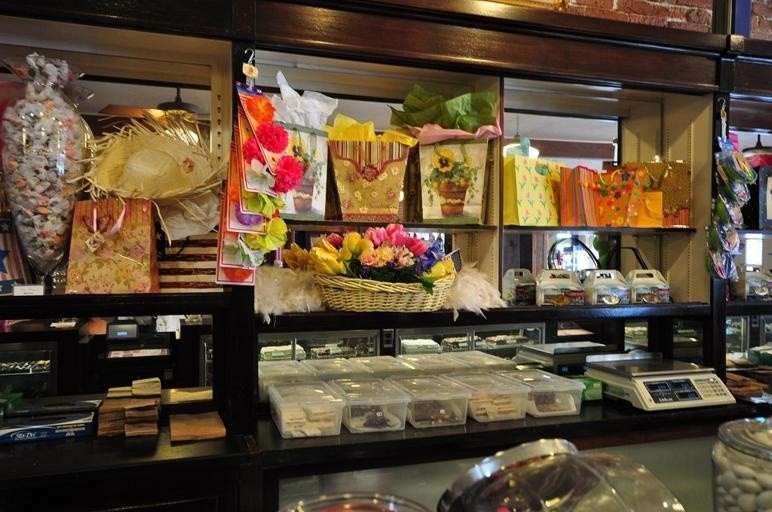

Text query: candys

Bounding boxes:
[2,53,85,259]
[712,430,772,512]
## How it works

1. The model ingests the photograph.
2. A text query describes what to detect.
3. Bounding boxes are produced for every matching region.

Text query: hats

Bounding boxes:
[91,134,213,199]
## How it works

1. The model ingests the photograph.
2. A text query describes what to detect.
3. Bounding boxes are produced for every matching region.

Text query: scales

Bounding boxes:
[585,356,737,411]
[511,342,619,375]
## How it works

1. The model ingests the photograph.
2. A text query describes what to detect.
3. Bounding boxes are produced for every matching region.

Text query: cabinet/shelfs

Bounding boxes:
[2,0,772,333]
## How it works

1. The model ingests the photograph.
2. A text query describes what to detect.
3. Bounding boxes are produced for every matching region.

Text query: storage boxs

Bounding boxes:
[389,376,471,429]
[495,368,585,418]
[268,382,345,434]
[440,373,530,422]
[327,379,410,434]
[253,349,517,401]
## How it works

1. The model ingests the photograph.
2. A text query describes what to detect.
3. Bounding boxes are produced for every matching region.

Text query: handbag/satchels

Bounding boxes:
[269,121,489,228]
[503,155,691,229]
[65,197,160,294]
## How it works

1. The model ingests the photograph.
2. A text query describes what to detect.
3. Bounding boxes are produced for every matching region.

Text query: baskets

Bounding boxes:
[311,269,455,313]
